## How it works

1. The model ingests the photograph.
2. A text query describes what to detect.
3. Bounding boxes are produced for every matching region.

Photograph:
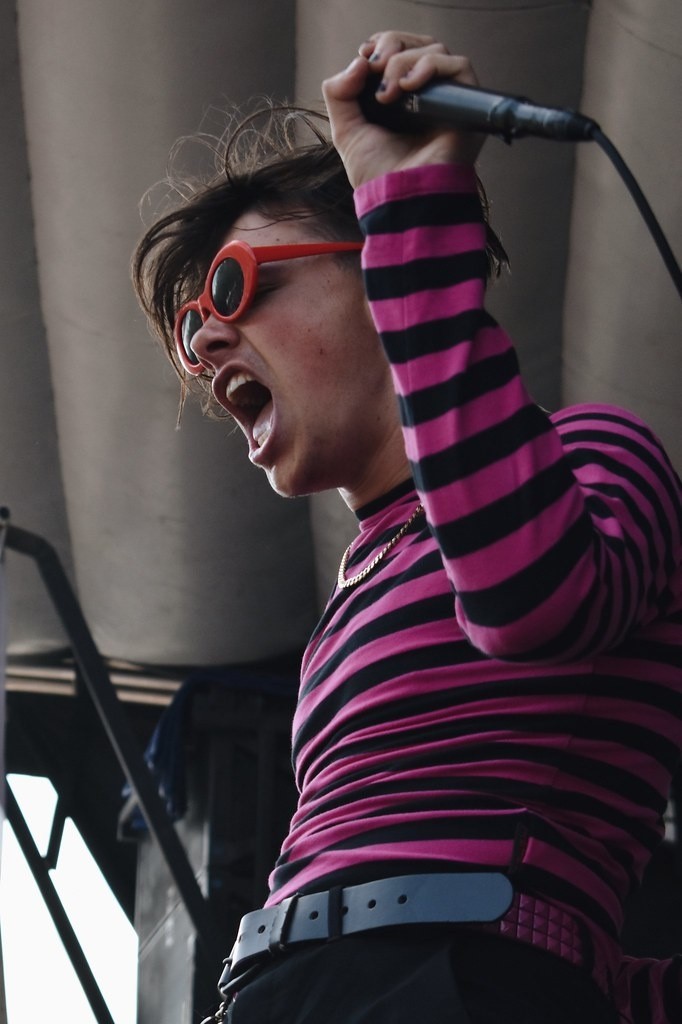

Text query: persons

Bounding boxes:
[131,28,682,1024]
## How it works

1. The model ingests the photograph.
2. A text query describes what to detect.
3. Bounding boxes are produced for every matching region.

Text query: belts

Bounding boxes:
[215,868,619,1002]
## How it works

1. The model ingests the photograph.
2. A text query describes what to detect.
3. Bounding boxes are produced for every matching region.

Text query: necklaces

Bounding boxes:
[337,502,423,588]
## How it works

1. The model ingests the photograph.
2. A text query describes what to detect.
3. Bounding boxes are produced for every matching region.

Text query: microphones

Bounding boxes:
[356,71,602,143]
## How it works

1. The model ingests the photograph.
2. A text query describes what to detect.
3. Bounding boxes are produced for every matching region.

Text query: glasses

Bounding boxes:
[174,240,363,377]
